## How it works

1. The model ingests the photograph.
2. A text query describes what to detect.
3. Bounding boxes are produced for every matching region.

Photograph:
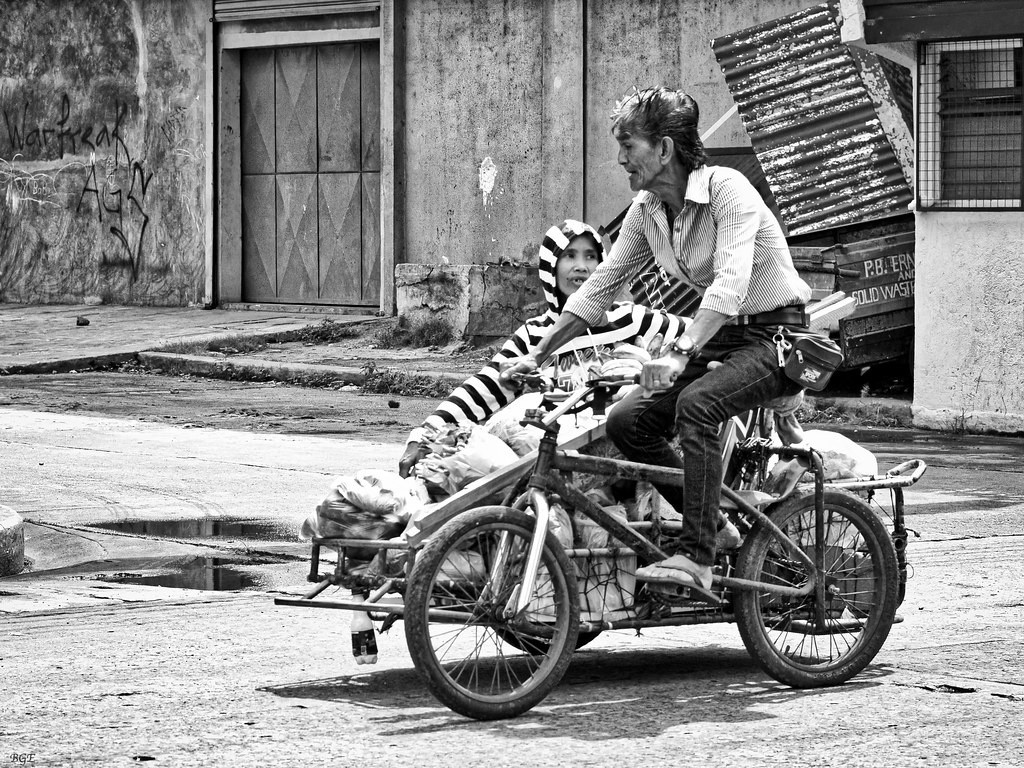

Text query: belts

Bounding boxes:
[723,303,812,329]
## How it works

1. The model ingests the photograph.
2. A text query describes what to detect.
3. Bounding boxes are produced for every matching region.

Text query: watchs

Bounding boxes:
[667,335,701,360]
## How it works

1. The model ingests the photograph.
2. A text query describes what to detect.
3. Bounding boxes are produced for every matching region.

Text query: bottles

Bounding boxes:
[350,587,378,665]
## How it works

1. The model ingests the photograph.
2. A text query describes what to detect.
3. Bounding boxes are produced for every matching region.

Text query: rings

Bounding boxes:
[653,379,660,383]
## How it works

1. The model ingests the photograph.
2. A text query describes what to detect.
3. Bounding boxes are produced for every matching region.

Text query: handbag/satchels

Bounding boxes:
[784,334,843,392]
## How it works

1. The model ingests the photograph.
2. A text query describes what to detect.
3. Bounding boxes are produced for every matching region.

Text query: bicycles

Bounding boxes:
[402,368,903,725]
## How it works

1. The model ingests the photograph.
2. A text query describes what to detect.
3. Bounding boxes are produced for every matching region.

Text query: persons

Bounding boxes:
[499,86,810,588]
[397,218,694,480]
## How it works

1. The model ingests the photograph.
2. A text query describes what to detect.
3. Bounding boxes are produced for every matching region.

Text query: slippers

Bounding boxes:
[636,559,721,604]
